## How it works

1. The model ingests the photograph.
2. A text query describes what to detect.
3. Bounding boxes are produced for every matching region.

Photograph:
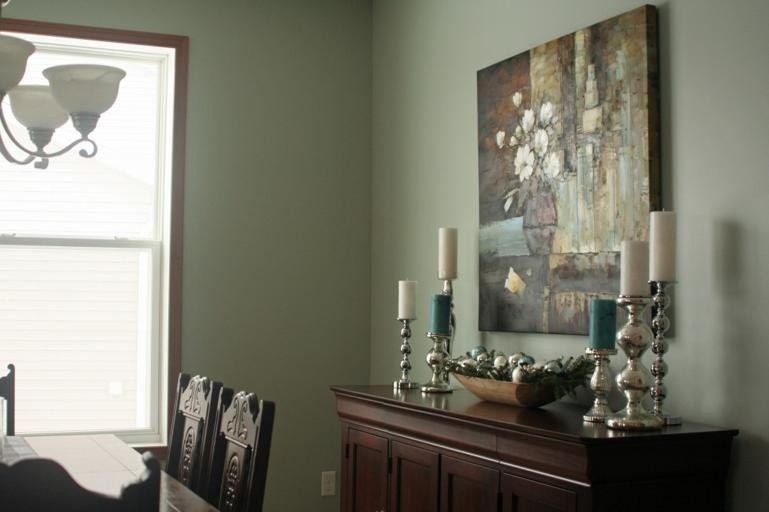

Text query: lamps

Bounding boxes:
[203,386,276,512]
[0,451,161,512]
[1,363,15,437]
[162,372,225,498]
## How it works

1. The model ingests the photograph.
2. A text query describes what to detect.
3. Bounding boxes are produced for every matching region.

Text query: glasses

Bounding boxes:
[2,2,128,171]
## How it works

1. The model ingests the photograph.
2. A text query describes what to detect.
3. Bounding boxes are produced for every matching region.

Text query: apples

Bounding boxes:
[437,226,460,279]
[431,295,451,335]
[588,297,617,350]
[650,207,680,282]
[619,239,652,299]
[397,278,418,319]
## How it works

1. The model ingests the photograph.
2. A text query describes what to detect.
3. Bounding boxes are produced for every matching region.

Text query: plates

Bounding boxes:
[328,382,740,511]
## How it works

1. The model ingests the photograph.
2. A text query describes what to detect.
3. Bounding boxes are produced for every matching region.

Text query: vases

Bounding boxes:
[494,85,572,215]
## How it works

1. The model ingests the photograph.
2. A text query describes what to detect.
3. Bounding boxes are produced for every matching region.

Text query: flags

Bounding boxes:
[523,185,557,255]
